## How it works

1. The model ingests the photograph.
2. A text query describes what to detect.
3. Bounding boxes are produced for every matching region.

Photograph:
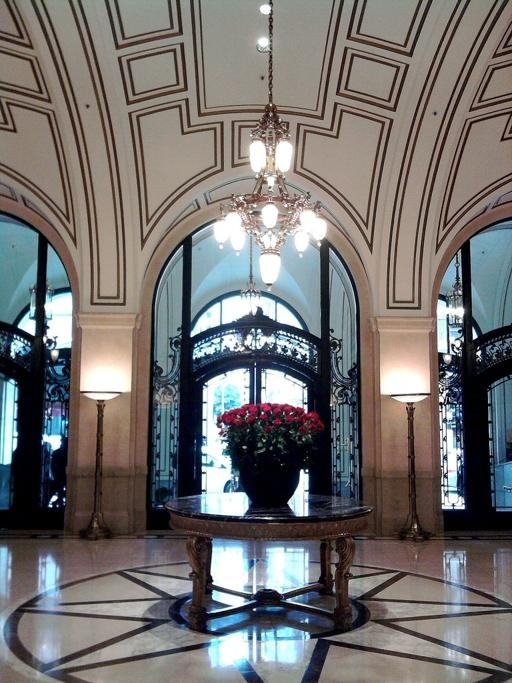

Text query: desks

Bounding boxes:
[164,490,375,633]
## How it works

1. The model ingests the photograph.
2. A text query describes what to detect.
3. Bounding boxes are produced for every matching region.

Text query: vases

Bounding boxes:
[237,462,300,507]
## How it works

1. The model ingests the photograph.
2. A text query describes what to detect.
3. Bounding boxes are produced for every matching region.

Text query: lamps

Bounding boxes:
[447,250,464,328]
[29,277,53,320]
[390,392,430,543]
[213,1,328,299]
[80,391,123,540]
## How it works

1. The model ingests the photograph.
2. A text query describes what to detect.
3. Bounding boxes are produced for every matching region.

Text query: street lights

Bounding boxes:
[390,393,433,542]
[79,390,122,541]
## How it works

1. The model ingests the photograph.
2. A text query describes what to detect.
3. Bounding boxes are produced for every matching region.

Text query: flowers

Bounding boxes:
[216,401,324,470]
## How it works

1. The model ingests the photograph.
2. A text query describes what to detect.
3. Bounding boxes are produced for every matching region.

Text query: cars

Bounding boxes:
[202,448,231,493]
